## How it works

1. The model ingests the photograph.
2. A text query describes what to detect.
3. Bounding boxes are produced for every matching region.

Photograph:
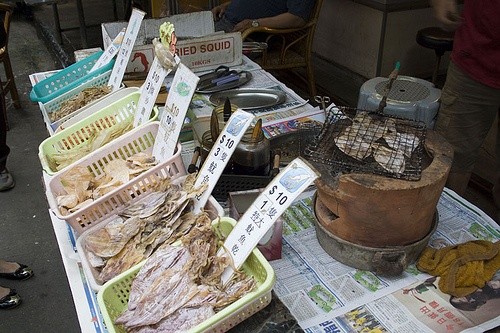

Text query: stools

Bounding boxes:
[356,74,441,129]
[414,27,455,84]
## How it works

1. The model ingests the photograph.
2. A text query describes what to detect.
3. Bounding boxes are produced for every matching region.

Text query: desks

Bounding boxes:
[29,55,500,333]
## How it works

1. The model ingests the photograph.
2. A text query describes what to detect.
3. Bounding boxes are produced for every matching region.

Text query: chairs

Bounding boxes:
[242,0,316,97]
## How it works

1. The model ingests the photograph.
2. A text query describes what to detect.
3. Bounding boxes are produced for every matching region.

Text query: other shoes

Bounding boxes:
[0,285,22,310]
[0,259,34,281]
[0,169,16,192]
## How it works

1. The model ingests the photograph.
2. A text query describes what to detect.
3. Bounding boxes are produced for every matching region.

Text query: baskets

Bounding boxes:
[29,50,115,103]
[97,215,275,333]
[76,174,225,292]
[46,121,187,235]
[43,70,125,125]
[38,91,160,177]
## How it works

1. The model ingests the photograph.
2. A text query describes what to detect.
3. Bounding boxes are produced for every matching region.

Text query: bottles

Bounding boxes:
[232,126,271,176]
[199,129,222,166]
[210,103,238,142]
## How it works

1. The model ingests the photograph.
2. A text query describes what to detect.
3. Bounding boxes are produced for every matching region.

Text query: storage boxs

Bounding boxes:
[102,11,242,73]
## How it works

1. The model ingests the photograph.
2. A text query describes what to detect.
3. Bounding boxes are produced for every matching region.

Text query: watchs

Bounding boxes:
[251,19,259,28]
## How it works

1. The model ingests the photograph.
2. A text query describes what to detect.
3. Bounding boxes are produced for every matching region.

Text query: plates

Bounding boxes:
[195,70,253,94]
[207,88,286,110]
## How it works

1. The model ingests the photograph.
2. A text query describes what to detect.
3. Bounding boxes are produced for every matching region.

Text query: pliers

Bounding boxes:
[314,95,330,125]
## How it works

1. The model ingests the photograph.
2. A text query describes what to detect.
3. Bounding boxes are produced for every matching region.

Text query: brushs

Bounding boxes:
[224,97,231,122]
[246,119,262,160]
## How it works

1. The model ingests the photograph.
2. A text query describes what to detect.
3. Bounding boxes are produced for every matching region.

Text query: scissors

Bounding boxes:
[198,65,237,85]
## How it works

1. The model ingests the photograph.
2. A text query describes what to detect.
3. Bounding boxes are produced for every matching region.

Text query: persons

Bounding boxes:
[0,10,15,193]
[432,0,500,198]
[211,0,317,61]
[0,260,33,310]
